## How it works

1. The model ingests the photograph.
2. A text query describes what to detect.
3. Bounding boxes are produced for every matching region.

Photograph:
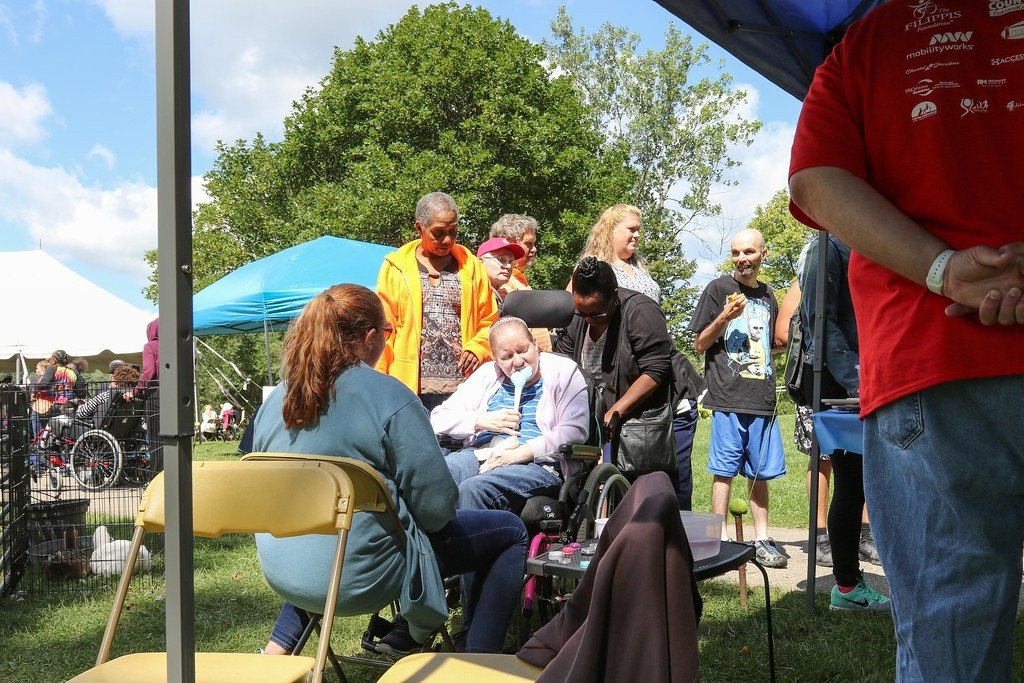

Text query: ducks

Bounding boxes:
[90,525,153,576]
[43,525,91,581]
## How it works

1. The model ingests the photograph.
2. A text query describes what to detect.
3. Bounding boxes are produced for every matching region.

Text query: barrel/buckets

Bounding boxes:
[28,498,91,547]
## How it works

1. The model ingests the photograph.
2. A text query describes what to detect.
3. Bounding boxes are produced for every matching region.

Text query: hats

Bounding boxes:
[477,237,525,259]
[108,360,125,373]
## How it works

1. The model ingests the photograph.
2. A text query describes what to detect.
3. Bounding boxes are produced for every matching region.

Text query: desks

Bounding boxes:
[526,539,775,683]
[809,409,863,454]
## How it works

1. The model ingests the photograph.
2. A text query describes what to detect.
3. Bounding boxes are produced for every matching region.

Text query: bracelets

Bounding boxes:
[925,250,955,296]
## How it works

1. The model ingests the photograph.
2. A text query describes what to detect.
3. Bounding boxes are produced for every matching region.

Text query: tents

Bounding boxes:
[192,236,399,387]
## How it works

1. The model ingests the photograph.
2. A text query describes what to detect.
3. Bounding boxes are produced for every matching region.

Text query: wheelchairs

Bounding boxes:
[214,419,240,441]
[437,369,633,649]
[28,386,155,490]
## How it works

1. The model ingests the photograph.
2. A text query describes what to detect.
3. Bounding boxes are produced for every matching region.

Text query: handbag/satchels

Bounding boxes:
[32,398,54,417]
[612,402,678,477]
[785,242,832,409]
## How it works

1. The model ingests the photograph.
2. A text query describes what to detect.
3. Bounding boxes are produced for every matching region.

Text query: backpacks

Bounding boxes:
[67,367,85,398]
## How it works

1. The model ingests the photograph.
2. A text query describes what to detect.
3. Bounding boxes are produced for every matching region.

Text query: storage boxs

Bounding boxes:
[672,510,723,560]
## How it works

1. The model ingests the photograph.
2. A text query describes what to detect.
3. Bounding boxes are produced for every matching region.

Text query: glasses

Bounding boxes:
[574,297,613,321]
[482,255,515,266]
[369,321,393,341]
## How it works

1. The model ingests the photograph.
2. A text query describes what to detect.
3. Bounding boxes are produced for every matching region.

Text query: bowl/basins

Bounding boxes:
[26,534,114,565]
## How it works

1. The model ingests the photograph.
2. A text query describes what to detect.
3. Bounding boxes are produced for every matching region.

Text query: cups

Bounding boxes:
[594,518,610,539]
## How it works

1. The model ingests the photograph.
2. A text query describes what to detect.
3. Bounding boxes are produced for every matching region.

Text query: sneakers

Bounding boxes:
[829,568,891,612]
[452,615,472,652]
[859,532,882,566]
[375,620,422,657]
[816,534,833,566]
[752,538,787,567]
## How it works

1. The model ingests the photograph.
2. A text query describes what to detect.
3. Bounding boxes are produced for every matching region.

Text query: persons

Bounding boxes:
[786,0,1023,681]
[489,213,554,354]
[564,203,662,307]
[477,236,525,312]
[0,318,161,487]
[797,236,893,615]
[251,283,529,656]
[361,315,590,658]
[557,255,699,519]
[371,192,501,413]
[687,226,787,569]
[201,402,243,442]
[774,276,883,567]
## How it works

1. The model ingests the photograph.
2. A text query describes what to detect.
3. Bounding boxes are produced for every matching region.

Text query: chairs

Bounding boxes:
[241,453,459,683]
[372,470,704,683]
[66,461,356,683]
